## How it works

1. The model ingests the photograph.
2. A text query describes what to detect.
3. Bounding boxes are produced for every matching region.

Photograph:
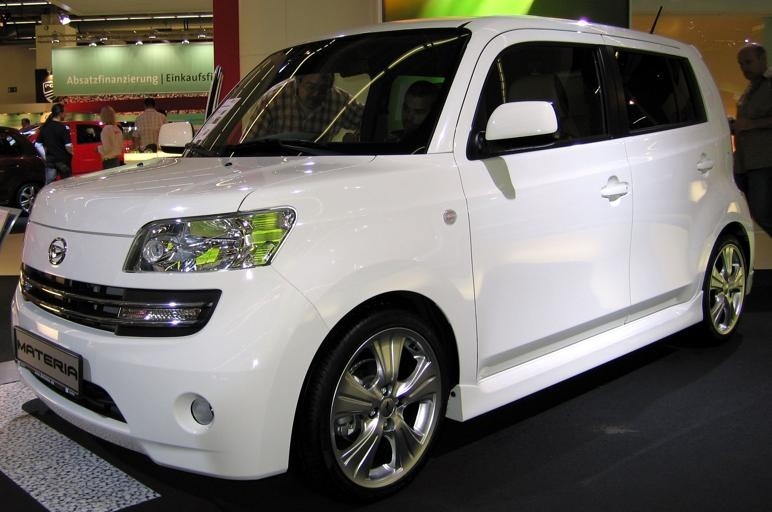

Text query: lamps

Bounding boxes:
[52,14,207,47]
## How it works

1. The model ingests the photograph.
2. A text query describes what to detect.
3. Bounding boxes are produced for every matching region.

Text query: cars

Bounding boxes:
[9,14,756,504]
[0,120,135,217]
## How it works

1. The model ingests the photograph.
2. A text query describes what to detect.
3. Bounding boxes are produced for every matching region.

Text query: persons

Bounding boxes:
[134,98,168,153]
[93,105,123,170]
[726,45,771,239]
[34,103,74,185]
[20,117,31,133]
[238,70,367,146]
[343,78,446,151]
